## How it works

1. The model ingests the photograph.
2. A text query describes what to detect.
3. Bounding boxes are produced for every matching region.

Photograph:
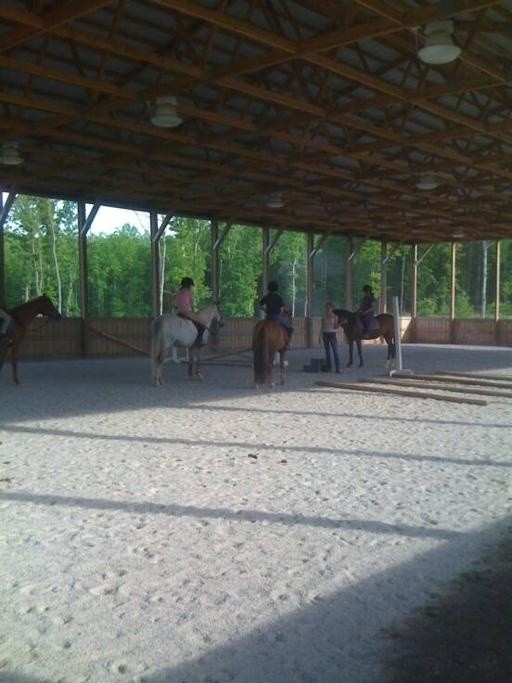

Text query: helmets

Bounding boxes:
[268,282,278,291]
[181,277,194,286]
[363,285,372,292]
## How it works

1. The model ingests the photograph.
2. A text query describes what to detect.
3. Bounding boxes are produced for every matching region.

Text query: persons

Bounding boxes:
[177,277,208,347]
[319,303,348,373]
[259,280,295,348]
[357,284,377,337]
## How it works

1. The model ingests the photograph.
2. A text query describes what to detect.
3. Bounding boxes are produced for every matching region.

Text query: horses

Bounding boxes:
[0,293,61,385]
[332,308,396,369]
[253,307,293,390]
[150,298,224,387]
